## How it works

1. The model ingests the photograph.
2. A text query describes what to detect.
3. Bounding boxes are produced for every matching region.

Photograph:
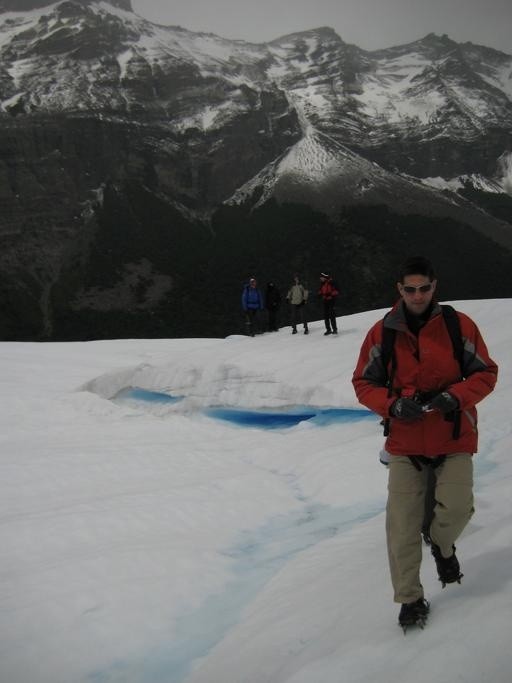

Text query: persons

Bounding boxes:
[316,272,339,335]
[243,273,310,337]
[351,257,498,625]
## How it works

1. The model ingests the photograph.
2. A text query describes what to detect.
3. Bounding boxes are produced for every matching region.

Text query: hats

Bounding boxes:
[320,271,330,279]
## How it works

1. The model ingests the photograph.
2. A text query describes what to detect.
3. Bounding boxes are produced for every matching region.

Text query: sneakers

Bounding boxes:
[292,329,308,334]
[430,542,464,588]
[398,598,430,636]
[324,328,338,335]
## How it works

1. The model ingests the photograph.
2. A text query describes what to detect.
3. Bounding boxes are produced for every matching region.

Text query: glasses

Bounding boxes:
[404,284,432,293]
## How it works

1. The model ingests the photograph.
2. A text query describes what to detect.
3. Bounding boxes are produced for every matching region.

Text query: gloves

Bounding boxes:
[390,392,458,420]
